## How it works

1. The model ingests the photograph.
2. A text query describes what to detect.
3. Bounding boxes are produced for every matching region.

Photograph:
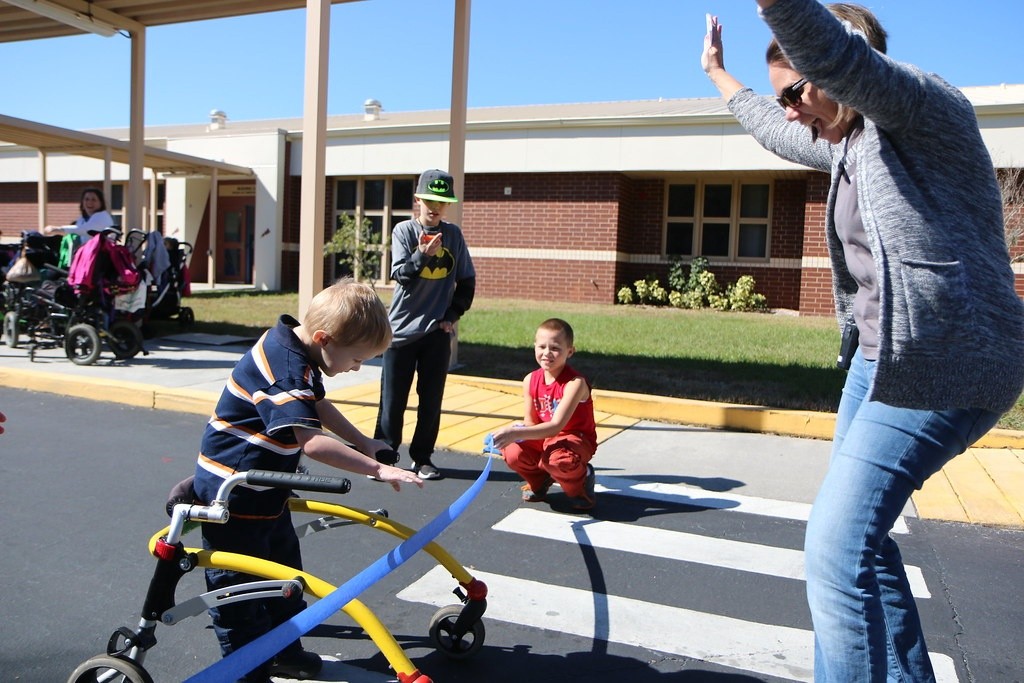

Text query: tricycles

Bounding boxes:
[64,467,489,683]
[0,227,150,368]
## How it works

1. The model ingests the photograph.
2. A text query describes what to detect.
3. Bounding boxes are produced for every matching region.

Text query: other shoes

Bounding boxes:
[270,650,324,679]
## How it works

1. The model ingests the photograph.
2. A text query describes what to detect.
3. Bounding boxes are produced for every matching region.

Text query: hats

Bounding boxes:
[415,168,459,203]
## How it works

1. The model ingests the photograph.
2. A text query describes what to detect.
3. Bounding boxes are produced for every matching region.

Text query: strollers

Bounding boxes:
[119,241,198,338]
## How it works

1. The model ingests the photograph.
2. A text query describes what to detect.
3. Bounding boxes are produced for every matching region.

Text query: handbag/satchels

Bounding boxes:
[7,244,40,282]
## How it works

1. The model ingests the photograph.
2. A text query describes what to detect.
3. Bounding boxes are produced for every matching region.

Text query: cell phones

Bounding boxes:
[423,235,440,244]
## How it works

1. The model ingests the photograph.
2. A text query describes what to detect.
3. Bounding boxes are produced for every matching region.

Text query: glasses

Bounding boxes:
[776,77,808,111]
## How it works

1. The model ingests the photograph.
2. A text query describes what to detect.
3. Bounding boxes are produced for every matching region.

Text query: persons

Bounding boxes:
[195,277,393,683]
[488,319,598,514]
[701,0,1024,683]
[44,187,115,244]
[367,169,476,479]
